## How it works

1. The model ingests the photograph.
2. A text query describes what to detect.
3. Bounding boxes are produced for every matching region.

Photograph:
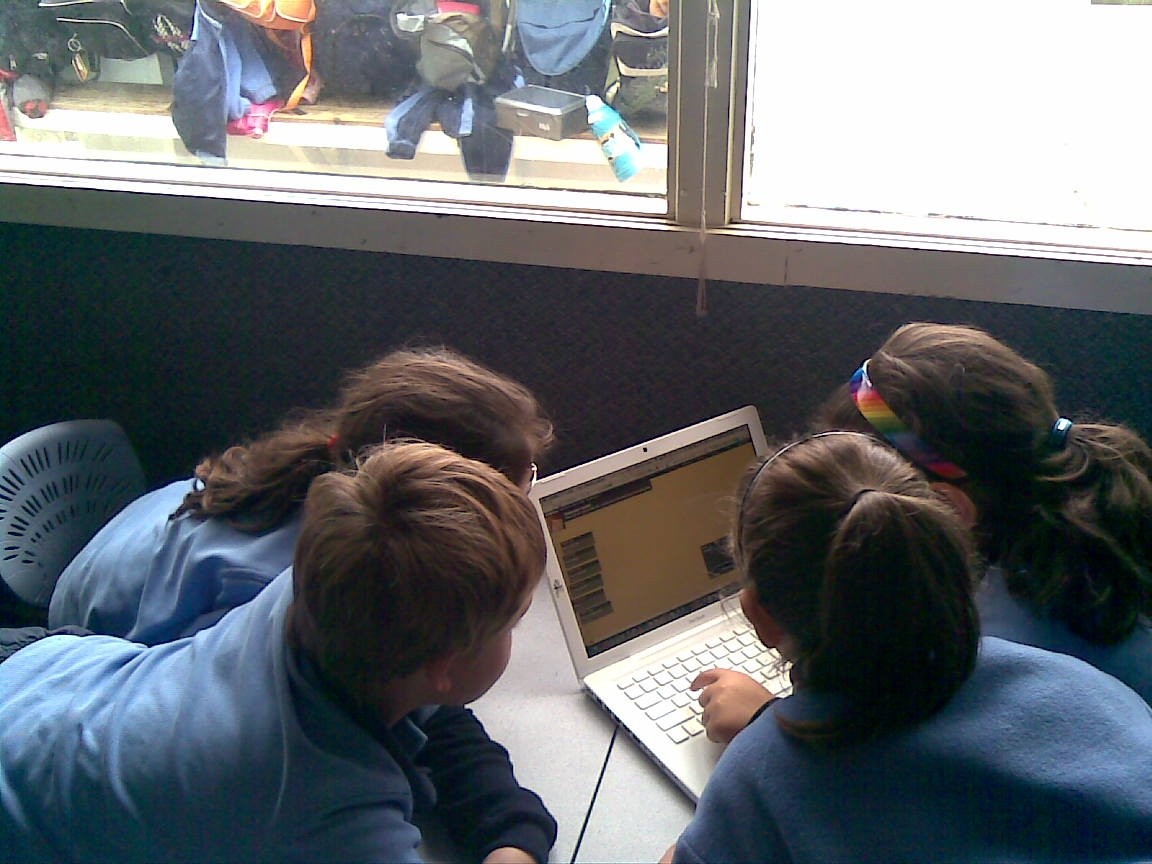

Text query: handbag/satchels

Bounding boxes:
[37,0,668,122]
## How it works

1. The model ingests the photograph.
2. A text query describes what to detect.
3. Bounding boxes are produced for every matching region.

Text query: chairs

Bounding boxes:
[0,419,147,605]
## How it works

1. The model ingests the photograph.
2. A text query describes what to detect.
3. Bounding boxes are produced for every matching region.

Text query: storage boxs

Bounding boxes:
[494,84,590,141]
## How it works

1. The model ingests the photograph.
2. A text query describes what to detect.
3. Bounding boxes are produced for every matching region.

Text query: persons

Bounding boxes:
[0,0,668,183]
[0,442,557,864]
[47,348,556,647]
[658,431,1152,864]
[822,324,1152,708]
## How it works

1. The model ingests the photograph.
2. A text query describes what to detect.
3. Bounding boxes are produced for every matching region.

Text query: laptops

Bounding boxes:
[526,403,796,807]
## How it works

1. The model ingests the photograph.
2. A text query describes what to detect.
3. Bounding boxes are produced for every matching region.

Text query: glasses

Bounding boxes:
[526,463,537,497]
